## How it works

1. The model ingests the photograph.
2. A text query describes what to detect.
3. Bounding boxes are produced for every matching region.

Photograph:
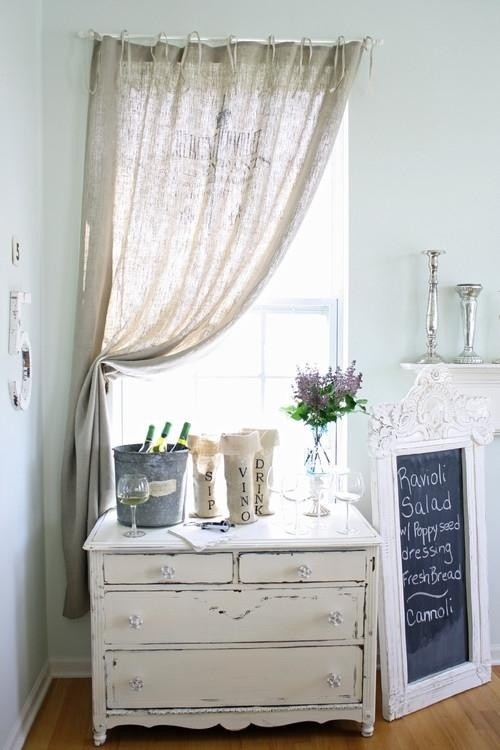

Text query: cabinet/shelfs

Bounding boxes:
[83,506,381,746]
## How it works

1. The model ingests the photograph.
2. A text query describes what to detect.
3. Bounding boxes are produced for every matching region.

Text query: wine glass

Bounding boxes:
[116,474,151,539]
[267,459,365,537]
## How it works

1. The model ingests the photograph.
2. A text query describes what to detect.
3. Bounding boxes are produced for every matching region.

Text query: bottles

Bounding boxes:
[135,422,193,453]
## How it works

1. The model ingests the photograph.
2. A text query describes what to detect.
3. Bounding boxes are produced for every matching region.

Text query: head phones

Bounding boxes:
[201,519,235,531]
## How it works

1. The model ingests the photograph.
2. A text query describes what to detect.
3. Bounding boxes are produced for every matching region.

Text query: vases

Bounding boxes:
[299,442,333,515]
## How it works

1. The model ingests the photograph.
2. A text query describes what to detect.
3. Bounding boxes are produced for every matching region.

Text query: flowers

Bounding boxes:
[287,359,369,515]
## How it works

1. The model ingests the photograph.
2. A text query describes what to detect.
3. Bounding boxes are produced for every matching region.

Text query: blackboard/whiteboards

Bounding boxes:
[372,438,493,722]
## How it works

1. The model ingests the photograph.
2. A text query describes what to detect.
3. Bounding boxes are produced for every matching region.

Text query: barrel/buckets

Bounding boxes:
[113,444,189,526]
